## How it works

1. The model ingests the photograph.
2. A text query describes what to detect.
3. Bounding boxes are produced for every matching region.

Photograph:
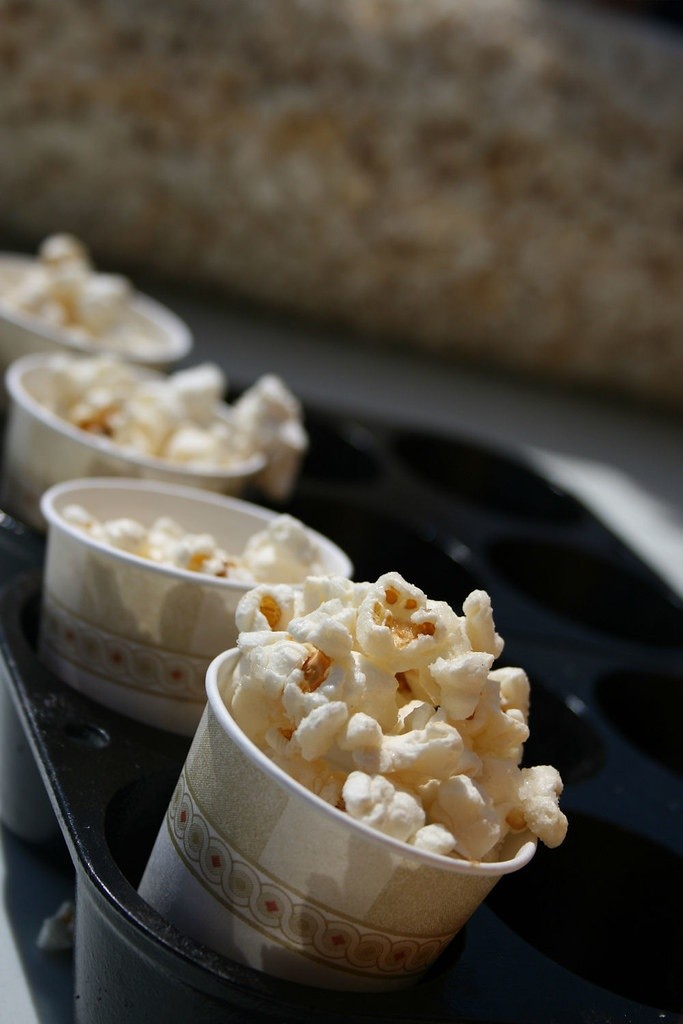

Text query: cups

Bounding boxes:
[137,649,538,1004]
[4,353,267,532]
[0,259,193,373]
[40,477,354,742]
